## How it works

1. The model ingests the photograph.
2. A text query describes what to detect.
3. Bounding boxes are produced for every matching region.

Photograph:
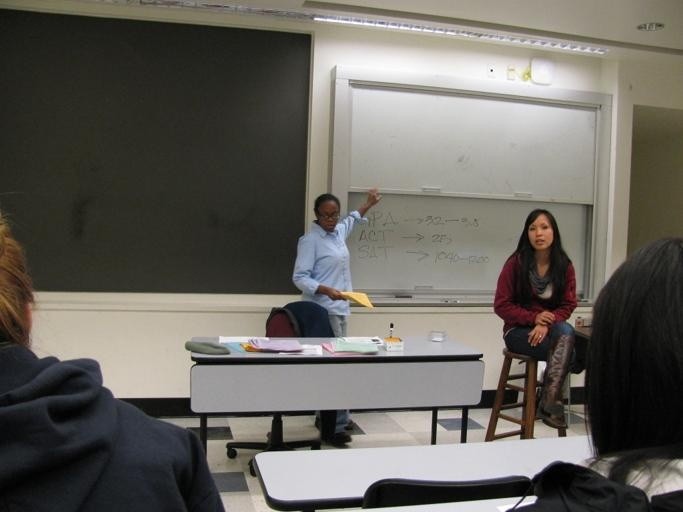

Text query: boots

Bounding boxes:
[535,333,576,429]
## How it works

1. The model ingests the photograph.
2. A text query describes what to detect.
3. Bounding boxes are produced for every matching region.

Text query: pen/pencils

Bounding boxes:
[390,323,393,338]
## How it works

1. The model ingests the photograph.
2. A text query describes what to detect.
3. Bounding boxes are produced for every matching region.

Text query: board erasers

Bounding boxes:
[395,296,412,298]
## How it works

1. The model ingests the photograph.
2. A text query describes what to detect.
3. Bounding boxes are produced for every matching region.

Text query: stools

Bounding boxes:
[484,348,567,441]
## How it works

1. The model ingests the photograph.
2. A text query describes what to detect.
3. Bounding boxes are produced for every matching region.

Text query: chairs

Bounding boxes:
[226,301,335,479]
[357,474,531,510]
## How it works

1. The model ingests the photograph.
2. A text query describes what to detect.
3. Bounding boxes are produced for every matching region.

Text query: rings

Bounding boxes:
[539,335,542,338]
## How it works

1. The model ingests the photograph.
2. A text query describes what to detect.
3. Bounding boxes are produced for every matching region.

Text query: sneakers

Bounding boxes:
[315,416,354,444]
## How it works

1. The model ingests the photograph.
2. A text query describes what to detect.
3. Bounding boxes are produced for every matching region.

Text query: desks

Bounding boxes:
[251,434,601,510]
[186,335,485,443]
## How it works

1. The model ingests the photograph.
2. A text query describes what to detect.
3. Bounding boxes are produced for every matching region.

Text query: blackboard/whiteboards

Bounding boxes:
[0,6,317,296]
[345,193,598,303]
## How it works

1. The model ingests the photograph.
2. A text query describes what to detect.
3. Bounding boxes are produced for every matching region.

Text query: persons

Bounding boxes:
[507,232,683,512]
[292,188,383,445]
[0,222,224,512]
[493,208,591,429]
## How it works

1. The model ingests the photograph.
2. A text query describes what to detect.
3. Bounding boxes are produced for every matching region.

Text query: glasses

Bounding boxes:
[316,209,340,220]
